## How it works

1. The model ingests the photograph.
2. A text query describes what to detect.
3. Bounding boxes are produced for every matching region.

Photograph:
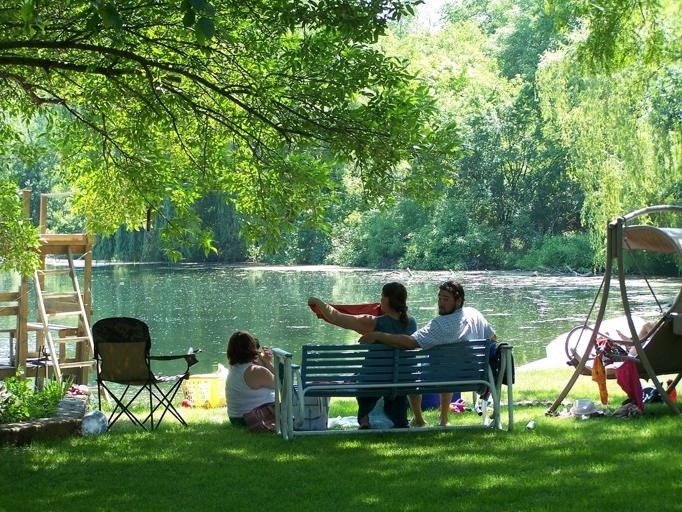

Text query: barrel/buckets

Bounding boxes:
[80,411,108,439]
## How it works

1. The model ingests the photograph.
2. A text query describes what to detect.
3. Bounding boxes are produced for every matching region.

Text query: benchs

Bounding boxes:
[270,343,514,440]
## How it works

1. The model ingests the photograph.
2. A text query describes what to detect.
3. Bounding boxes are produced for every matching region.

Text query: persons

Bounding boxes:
[362,279,499,423]
[225,332,280,435]
[612,323,663,353]
[309,284,419,429]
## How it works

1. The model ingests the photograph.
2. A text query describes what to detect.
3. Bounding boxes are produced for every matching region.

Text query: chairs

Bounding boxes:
[91,317,202,433]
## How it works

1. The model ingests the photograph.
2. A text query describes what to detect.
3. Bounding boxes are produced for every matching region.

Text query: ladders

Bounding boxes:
[29,246,110,407]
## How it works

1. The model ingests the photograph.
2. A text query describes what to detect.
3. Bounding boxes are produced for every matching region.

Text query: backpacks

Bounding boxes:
[289,380,333,431]
